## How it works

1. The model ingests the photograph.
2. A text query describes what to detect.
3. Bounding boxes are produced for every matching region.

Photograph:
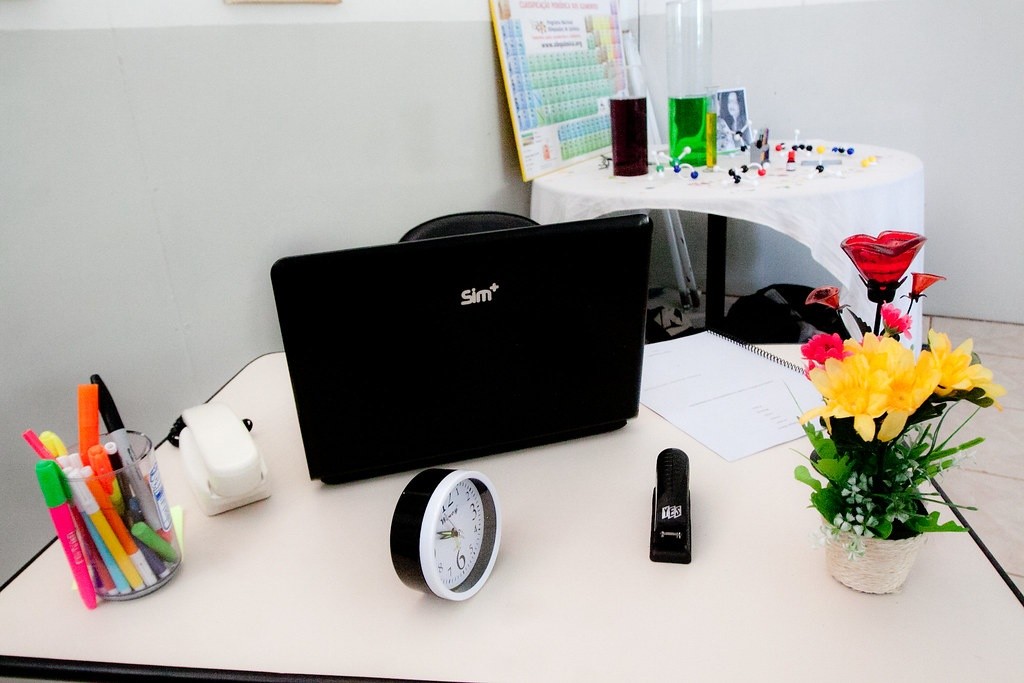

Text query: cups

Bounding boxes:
[51,429,181,599]
[670,96,708,166]
[611,98,649,177]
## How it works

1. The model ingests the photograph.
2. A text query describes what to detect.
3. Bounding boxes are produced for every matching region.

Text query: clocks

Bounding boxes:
[390,469,500,601]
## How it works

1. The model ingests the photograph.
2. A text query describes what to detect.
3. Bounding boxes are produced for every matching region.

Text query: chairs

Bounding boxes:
[396,211,539,244]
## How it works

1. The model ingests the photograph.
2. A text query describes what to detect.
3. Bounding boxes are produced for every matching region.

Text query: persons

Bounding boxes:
[719,90,751,147]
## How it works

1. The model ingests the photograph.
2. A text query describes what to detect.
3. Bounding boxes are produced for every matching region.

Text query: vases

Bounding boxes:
[822,490,929,593]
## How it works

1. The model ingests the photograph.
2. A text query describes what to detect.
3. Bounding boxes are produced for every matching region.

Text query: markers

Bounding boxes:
[21,372,182,609]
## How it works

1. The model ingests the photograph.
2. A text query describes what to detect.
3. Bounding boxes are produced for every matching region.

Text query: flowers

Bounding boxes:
[793,230,1004,539]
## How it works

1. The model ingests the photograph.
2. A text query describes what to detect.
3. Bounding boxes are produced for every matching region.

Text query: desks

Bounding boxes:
[0,341,1024,683]
[530,141,922,360]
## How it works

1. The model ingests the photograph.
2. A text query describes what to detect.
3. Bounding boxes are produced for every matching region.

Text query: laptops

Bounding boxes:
[271,214,654,484]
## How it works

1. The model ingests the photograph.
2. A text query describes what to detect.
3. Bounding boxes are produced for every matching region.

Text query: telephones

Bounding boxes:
[180,405,271,518]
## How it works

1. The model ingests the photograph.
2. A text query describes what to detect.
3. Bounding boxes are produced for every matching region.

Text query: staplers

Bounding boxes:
[649,446,693,564]
